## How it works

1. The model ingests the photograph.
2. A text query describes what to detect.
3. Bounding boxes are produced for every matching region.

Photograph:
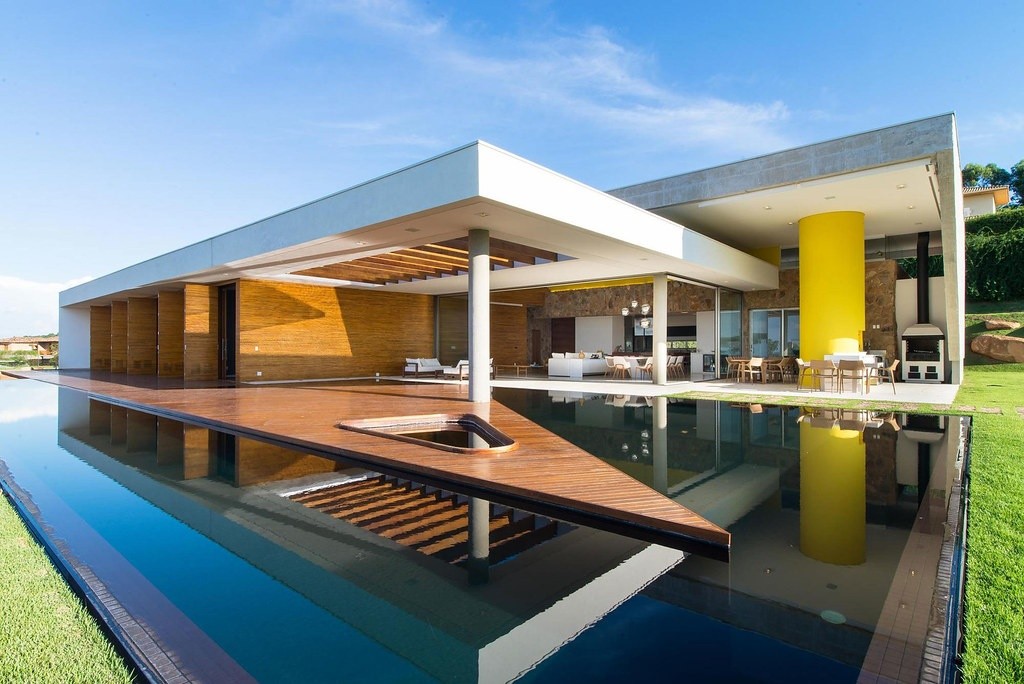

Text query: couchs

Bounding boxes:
[547,390,602,403]
[548,352,605,376]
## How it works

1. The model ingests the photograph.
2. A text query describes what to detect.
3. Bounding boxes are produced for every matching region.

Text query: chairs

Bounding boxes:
[604,355,900,396]
[604,394,683,407]
[730,401,900,431]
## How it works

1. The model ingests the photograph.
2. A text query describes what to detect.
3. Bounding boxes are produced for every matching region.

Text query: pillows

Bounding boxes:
[424,359,439,365]
[456,359,469,368]
[551,353,563,358]
[405,357,421,367]
[490,357,493,366]
[565,397,578,402]
[551,397,564,402]
[565,352,577,358]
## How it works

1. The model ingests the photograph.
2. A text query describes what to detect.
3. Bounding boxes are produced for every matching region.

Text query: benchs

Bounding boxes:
[402,357,494,382]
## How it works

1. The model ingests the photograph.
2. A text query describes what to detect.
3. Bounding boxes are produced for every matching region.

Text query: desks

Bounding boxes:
[731,360,779,384]
[604,357,653,380]
[605,394,646,406]
[804,407,883,428]
[731,402,750,407]
[812,362,883,394]
[495,364,531,377]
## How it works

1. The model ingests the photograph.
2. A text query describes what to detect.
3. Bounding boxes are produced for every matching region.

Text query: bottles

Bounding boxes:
[579,350,585,359]
